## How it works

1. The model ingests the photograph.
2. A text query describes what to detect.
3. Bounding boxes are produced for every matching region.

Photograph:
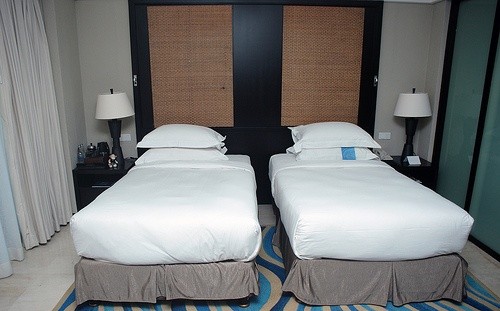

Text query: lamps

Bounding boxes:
[91,88,133,169]
[394,86,433,159]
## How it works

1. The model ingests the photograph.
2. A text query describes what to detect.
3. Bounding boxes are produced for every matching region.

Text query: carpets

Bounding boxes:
[51,226,500,311]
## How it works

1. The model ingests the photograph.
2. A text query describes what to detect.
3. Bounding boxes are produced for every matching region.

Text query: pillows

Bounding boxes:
[287,122,383,149]
[137,125,226,150]
[290,146,379,165]
[135,146,227,167]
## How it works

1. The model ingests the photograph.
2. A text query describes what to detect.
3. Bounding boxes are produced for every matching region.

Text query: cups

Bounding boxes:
[108,156,119,168]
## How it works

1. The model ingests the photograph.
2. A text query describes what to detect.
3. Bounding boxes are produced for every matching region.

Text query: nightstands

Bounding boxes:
[380,155,437,190]
[71,156,139,213]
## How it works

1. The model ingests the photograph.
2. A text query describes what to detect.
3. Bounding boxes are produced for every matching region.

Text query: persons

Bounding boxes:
[108,154,118,168]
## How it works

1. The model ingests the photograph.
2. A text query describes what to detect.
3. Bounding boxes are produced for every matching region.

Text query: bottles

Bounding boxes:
[78,142,109,164]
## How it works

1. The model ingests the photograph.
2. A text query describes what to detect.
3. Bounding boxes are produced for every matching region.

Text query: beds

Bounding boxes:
[70,153,262,303]
[268,155,475,306]
